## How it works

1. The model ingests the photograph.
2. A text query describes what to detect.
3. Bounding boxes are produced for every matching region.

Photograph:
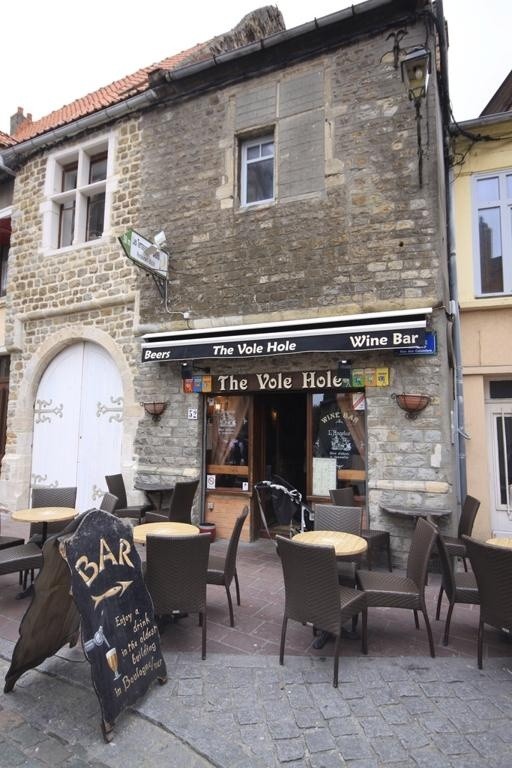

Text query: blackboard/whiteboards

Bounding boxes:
[4,508,98,693]
[58,509,168,743]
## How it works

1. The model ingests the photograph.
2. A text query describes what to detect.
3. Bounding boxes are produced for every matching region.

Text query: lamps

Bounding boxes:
[179,361,210,374]
[139,401,171,421]
[338,359,351,378]
[391,391,430,420]
[400,45,433,189]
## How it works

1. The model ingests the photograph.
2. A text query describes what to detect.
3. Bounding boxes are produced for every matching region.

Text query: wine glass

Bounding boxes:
[104,648,122,682]
